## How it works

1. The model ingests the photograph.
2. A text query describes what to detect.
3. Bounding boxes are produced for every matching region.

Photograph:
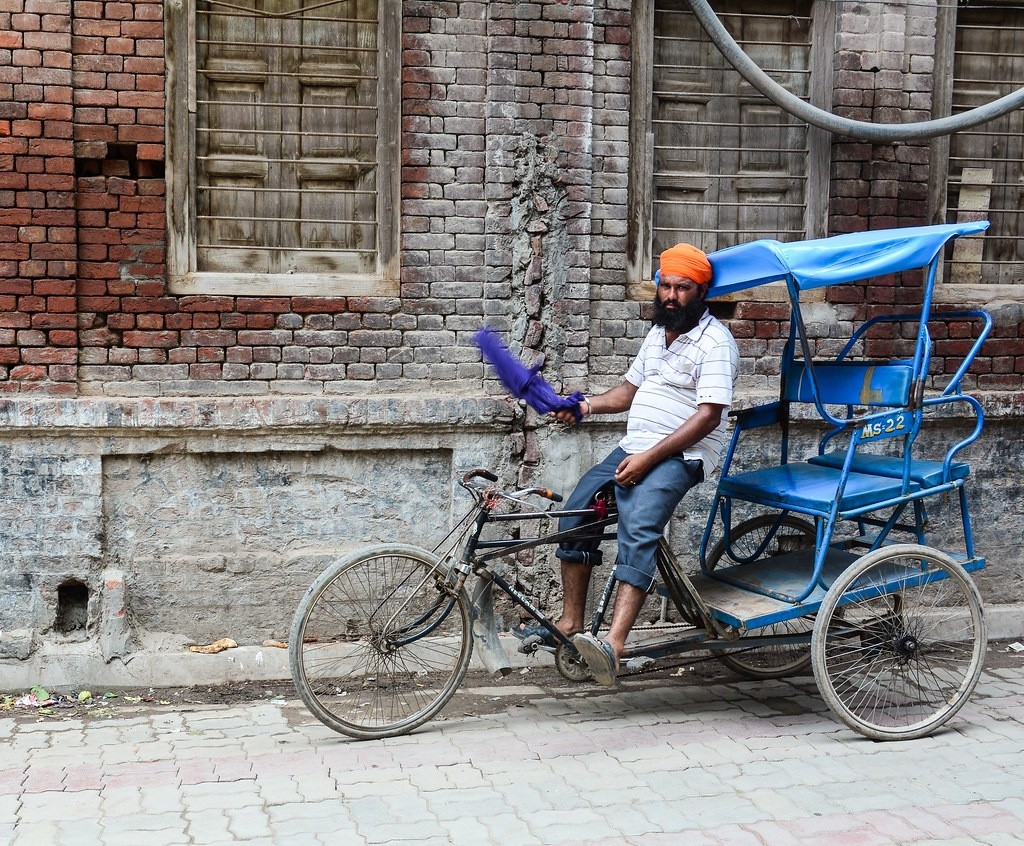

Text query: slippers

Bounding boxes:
[574,632,618,685]
[510,624,557,654]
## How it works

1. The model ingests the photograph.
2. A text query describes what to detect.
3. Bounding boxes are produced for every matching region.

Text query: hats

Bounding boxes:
[660,243,713,284]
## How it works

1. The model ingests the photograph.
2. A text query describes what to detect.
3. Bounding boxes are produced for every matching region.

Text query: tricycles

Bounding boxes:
[292,219,995,742]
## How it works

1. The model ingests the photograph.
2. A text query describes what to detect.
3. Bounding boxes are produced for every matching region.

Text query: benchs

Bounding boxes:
[718,358,980,592]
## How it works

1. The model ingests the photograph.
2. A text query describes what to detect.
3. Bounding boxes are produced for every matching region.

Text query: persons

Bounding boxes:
[510,244,739,684]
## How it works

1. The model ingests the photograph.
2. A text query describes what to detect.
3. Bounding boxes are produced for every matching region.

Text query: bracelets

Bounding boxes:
[584,396,591,414]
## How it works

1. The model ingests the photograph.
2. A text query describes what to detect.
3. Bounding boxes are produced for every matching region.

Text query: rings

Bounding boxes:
[631,481,636,485]
